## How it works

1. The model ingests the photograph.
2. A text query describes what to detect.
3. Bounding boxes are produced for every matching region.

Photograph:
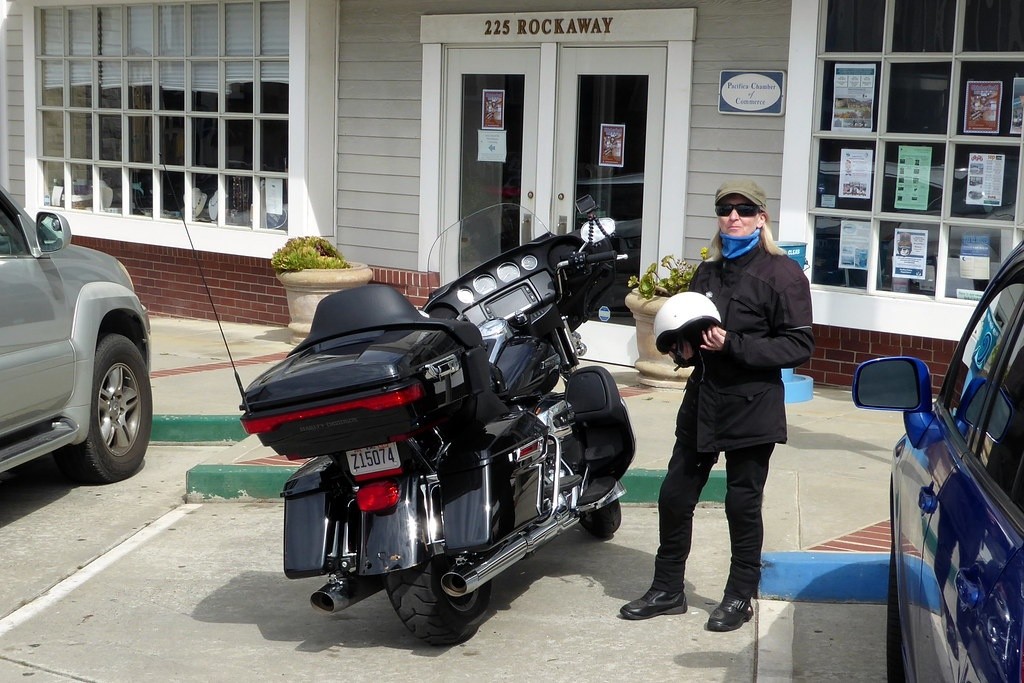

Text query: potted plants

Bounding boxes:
[271,236,375,345]
[625,247,709,388]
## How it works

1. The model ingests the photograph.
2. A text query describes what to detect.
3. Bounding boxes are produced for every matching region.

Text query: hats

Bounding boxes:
[714,177,767,212]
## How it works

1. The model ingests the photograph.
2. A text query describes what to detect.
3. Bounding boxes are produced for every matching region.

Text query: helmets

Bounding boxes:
[653,291,721,356]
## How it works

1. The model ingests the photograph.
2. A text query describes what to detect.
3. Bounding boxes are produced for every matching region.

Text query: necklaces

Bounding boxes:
[191,197,201,214]
[210,196,218,207]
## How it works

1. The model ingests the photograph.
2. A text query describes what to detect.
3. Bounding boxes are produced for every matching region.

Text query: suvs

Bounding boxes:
[0,184,154,487]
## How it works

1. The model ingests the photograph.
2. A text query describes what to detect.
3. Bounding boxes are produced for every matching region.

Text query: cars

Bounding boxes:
[849,237,1024,683]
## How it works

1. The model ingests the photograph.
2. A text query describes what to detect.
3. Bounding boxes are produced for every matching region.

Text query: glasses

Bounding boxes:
[715,203,764,217]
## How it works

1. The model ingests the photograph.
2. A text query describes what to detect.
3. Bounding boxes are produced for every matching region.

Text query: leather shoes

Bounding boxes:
[707,595,754,632]
[620,587,687,620]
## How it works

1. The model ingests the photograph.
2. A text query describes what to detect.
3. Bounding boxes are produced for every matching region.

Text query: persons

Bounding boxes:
[621,178,814,633]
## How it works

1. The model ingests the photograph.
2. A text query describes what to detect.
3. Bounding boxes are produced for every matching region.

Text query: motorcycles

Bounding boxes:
[221,193,637,648]
